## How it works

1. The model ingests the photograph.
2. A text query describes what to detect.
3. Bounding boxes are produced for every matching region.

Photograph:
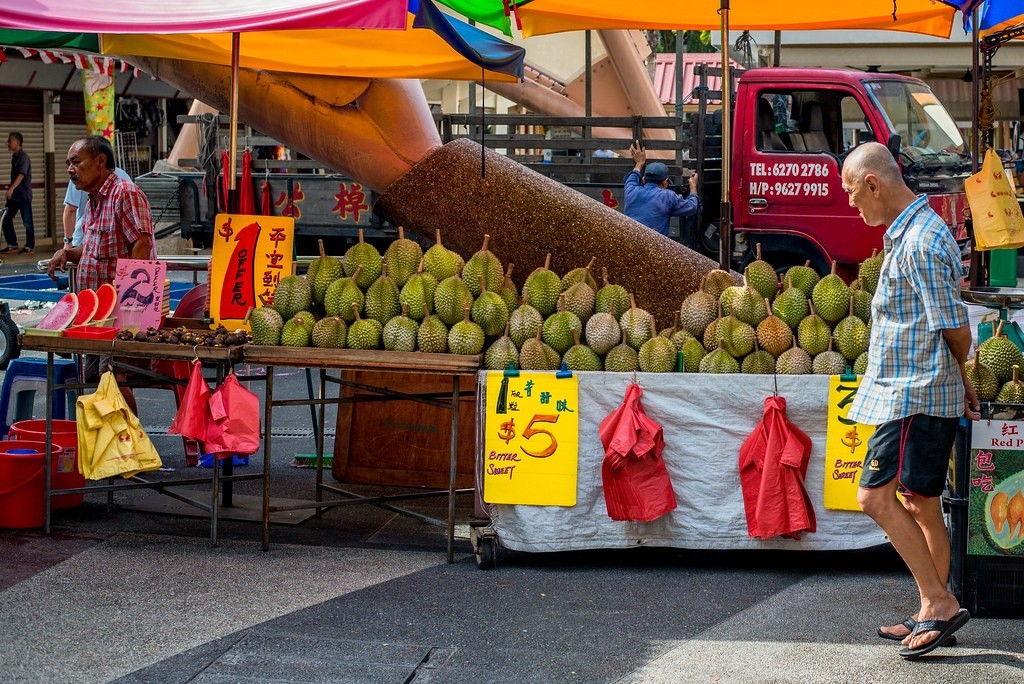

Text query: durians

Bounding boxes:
[243,224,884,374]
[962,317,1024,405]
[969,448,1024,558]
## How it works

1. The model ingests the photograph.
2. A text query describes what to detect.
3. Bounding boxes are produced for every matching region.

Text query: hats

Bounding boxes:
[644,162,673,185]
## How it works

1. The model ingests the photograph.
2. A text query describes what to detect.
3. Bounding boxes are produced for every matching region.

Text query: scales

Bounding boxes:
[959,288,1024,354]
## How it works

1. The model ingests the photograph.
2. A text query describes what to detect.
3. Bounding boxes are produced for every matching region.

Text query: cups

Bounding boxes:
[122,306,143,337]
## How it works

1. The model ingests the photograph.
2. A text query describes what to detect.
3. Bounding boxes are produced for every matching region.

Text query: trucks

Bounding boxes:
[152,64,974,289]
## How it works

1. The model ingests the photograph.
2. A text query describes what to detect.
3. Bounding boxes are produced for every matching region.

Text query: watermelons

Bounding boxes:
[35,282,117,330]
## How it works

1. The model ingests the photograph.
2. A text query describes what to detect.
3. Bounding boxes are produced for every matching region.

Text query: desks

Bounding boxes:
[19,331,254,546]
[476,367,890,567]
[242,340,484,563]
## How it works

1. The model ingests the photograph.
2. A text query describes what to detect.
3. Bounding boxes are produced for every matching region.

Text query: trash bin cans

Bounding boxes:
[990,248,1017,287]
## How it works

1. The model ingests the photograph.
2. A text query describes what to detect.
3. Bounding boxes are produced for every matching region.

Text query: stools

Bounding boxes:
[0,357,81,440]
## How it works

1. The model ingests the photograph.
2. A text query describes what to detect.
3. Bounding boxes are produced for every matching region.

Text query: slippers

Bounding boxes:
[899,608,970,657]
[878,617,956,648]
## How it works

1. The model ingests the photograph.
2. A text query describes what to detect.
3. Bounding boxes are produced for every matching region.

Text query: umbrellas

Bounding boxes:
[0,0,1024,508]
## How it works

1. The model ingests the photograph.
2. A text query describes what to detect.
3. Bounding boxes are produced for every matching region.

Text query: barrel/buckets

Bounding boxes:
[7,418,85,507]
[0,440,64,531]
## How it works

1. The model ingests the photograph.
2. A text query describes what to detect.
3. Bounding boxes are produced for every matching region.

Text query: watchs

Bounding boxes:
[64,237,73,244]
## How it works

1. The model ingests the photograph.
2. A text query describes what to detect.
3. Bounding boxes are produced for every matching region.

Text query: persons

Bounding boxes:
[841,141,981,657]
[47,136,157,417]
[63,167,133,249]
[592,149,613,157]
[0,132,36,255]
[623,140,699,236]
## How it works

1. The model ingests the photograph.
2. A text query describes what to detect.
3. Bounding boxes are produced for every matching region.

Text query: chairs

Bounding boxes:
[799,101,831,151]
[758,98,787,150]
[783,132,806,151]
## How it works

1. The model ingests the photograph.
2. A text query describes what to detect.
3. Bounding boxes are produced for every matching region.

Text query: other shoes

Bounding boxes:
[0,247,19,254]
[19,246,34,254]
